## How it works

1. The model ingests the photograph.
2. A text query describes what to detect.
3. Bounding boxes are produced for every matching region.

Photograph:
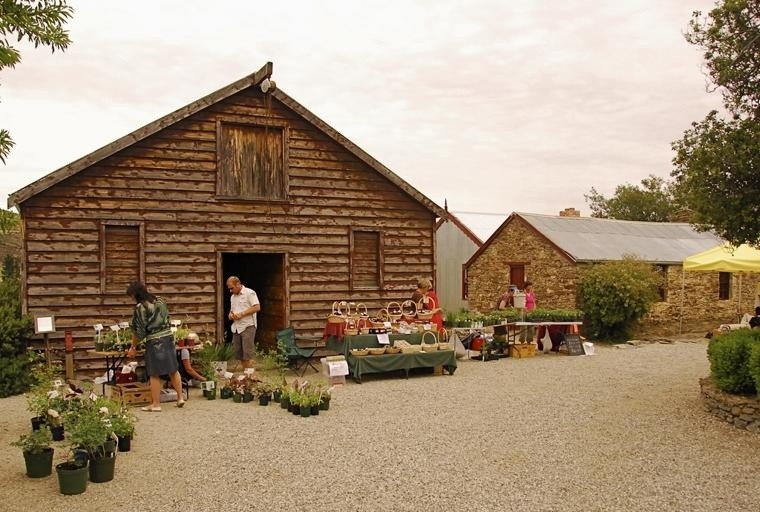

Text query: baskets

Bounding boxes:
[386,296,436,321]
[348,348,370,357]
[420,331,439,352]
[437,327,454,351]
[384,346,401,354]
[326,300,371,336]
[367,308,389,328]
[366,347,386,355]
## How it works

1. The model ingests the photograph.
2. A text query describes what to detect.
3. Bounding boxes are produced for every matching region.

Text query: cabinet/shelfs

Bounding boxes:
[326,318,456,383]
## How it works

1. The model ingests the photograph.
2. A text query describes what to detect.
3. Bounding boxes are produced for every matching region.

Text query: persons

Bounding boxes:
[749,306,760,329]
[411,279,442,319]
[160,333,207,388]
[226,276,261,369]
[495,288,515,335]
[127,282,185,413]
[519,282,537,344]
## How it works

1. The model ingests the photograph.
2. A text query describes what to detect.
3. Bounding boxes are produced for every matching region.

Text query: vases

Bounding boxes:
[23,448,54,477]
[56,461,89,495]
[89,454,117,483]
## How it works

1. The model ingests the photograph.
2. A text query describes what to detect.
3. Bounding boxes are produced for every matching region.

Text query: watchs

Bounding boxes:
[131,345,137,350]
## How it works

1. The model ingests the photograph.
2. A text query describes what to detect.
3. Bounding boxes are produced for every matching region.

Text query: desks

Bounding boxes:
[493,320,583,343]
[86,342,198,401]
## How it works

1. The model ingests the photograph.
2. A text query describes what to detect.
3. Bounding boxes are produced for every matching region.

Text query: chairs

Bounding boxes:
[453,327,497,362]
[275,326,319,378]
[753,326,760,329]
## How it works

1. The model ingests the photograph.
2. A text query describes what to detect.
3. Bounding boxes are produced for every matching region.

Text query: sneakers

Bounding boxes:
[140,405,163,412]
[174,400,186,408]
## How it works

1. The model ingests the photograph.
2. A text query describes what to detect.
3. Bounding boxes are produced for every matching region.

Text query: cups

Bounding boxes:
[471,320,483,330]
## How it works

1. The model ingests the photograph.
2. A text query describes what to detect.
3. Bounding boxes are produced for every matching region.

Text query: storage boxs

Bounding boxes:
[508,343,536,358]
[110,382,153,405]
[713,329,722,337]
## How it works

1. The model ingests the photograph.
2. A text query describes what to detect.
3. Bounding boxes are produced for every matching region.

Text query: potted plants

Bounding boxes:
[446,307,520,328]
[199,343,331,417]
[10,347,135,460]
[92,314,200,347]
[175,323,190,346]
